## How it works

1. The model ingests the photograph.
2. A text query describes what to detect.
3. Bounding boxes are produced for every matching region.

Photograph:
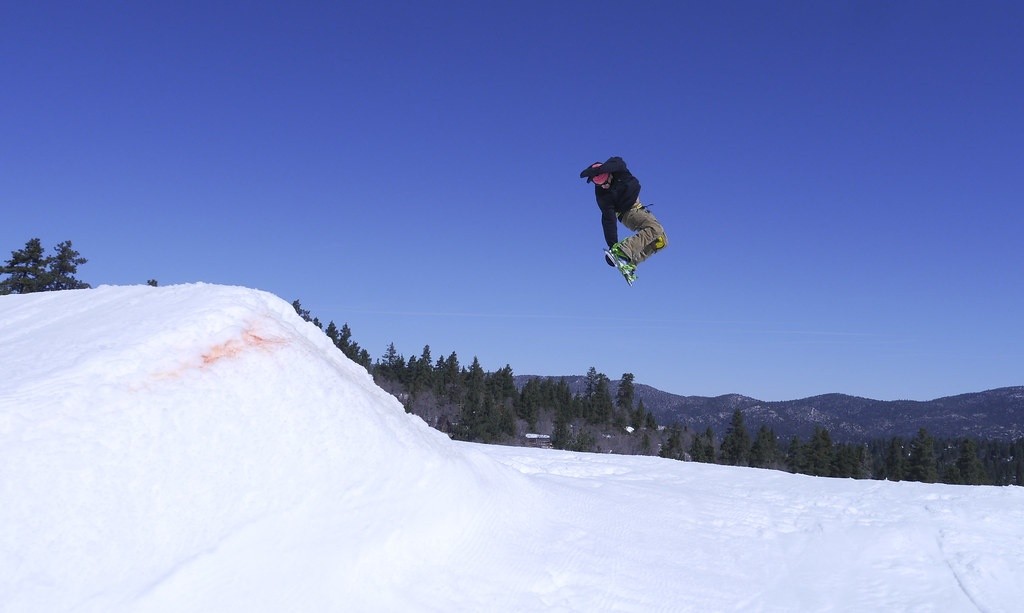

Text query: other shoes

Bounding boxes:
[609,243,632,275]
[623,264,638,286]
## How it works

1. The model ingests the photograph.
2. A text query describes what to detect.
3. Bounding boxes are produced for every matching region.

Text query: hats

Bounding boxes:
[592,164,609,184]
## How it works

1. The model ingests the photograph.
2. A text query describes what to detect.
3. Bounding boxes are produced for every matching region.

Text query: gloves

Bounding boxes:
[605,255,616,267]
[580,167,603,184]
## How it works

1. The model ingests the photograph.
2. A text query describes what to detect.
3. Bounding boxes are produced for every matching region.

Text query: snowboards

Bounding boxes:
[602,230,668,288]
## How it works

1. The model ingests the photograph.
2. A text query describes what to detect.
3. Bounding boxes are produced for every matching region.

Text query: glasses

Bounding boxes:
[594,175,610,187]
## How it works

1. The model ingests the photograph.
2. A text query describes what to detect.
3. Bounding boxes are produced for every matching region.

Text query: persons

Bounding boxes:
[580,157,667,284]
